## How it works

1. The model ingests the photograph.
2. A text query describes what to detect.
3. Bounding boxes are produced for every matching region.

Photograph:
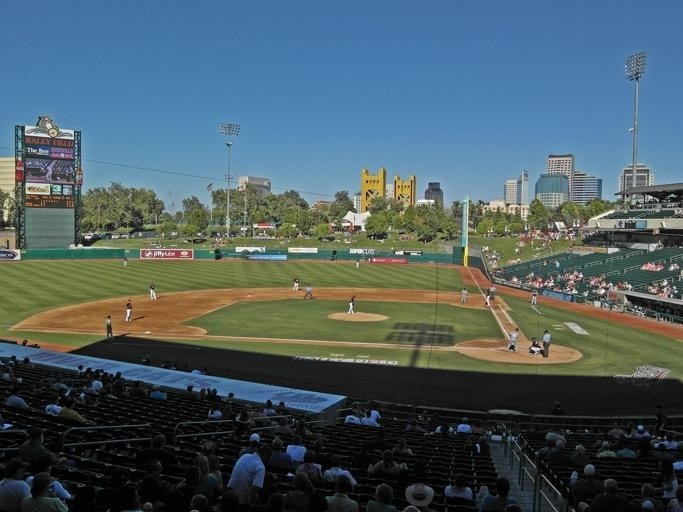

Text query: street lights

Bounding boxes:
[618,47,647,189]
[215,120,240,237]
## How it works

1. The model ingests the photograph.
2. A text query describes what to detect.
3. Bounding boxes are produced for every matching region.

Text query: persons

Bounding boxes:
[347,295,355,314]
[149,282,157,300]
[482,230,683,313]
[124,298,132,322]
[507,329,550,357]
[25,159,75,183]
[292,277,300,291]
[625,196,683,217]
[461,287,467,304]
[355,257,361,269]
[303,284,314,301]
[1,339,517,510]
[538,401,682,510]
[106,315,114,343]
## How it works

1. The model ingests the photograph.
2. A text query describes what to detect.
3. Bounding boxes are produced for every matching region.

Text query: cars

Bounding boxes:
[84,232,130,240]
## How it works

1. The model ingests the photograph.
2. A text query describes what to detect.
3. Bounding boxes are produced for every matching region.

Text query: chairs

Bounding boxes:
[0,359,683,512]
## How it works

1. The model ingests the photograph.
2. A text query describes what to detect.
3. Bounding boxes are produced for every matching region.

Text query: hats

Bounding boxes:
[250,433,260,442]
[405,482,435,507]
[261,445,274,456]
[34,472,59,481]
